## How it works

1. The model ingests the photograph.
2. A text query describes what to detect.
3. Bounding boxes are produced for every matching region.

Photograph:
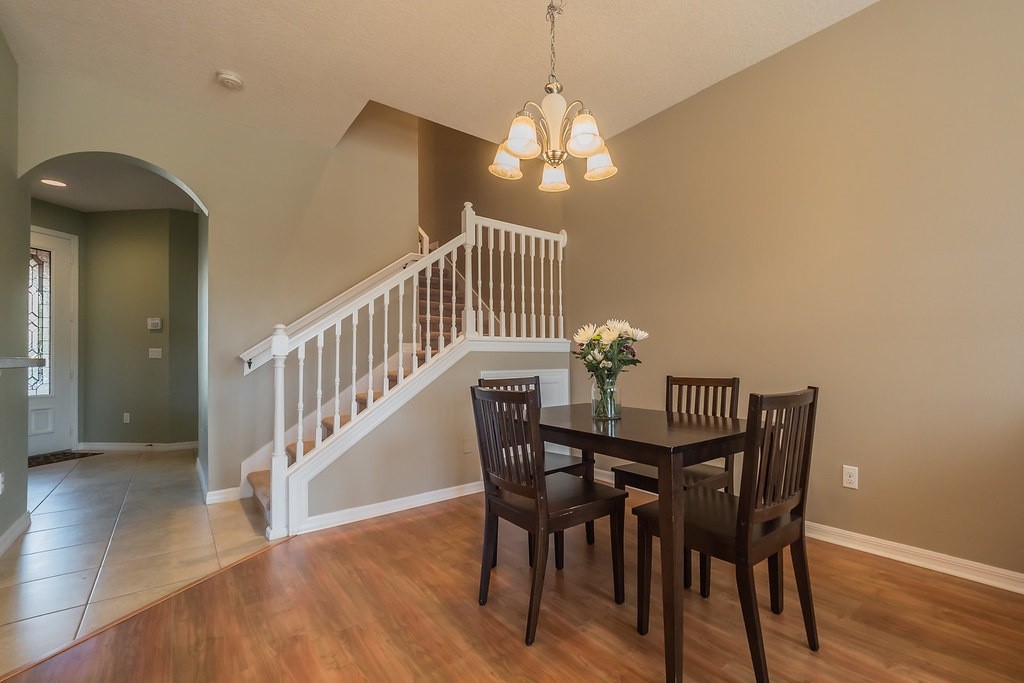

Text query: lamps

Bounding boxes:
[488,0,619,193]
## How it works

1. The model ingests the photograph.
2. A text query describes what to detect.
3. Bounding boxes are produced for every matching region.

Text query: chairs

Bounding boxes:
[477,375,596,566]
[611,375,740,589]
[633,386,820,683]
[469,386,630,646]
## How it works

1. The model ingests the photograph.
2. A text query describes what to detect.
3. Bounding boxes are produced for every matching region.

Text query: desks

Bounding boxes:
[485,402,784,683]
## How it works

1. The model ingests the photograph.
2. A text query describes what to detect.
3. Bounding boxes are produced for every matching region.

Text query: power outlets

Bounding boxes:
[123,413,130,423]
[841,465,858,491]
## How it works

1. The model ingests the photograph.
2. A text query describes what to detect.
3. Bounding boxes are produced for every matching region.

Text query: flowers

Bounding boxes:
[570,319,649,371]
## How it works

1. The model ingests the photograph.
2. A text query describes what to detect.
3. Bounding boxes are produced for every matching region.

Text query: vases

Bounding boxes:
[590,370,622,421]
